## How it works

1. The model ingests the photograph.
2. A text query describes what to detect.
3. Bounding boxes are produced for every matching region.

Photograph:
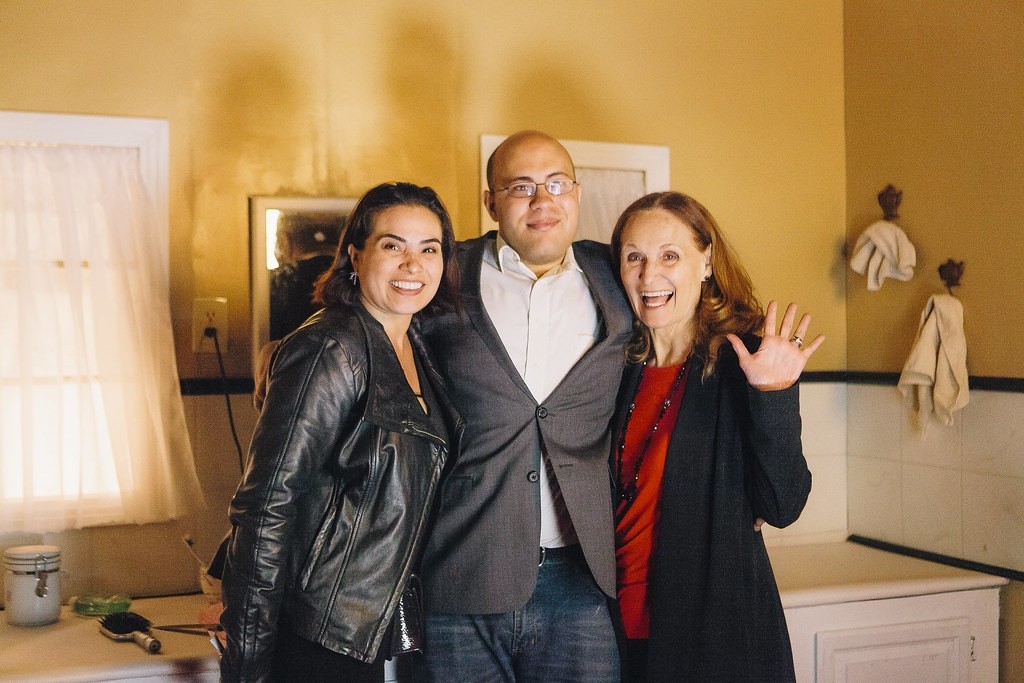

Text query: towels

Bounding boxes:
[897,294,970,436]
[850,219,916,291]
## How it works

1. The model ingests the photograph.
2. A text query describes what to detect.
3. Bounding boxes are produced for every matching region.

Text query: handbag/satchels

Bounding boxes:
[389,573,427,657]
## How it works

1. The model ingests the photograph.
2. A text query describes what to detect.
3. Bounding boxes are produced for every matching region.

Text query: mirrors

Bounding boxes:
[481,135,673,243]
[247,196,353,381]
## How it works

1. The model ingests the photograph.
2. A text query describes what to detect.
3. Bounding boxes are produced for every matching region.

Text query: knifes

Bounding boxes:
[152,623,225,631]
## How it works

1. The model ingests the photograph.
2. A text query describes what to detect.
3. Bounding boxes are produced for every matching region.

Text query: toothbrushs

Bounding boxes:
[183,536,205,567]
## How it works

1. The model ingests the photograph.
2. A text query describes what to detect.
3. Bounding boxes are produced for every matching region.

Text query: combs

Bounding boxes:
[97,611,162,654]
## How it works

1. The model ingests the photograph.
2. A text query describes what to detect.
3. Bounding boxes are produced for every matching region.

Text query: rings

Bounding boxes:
[789,334,803,348]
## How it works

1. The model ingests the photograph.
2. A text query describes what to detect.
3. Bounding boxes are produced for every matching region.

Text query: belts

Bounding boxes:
[539,544,582,568]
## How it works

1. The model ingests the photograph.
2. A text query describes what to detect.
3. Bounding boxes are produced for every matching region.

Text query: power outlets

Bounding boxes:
[192,297,229,355]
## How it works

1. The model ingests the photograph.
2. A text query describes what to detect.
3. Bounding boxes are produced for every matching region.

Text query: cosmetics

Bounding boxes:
[67,592,132,615]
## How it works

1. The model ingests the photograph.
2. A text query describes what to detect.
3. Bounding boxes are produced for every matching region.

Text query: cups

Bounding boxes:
[200,566,222,605]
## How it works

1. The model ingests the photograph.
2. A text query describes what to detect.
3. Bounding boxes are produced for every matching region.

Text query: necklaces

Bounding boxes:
[616,350,691,503]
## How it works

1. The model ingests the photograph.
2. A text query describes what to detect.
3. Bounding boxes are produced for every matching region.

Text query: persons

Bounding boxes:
[253,130,635,683]
[218,184,463,683]
[610,191,826,683]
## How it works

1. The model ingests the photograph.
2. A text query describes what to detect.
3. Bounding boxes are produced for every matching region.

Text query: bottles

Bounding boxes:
[68,593,132,615]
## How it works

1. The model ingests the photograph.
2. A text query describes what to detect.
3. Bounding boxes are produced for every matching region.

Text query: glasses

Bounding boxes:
[491,177,581,198]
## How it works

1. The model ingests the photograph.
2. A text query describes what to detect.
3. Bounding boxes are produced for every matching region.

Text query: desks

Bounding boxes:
[2,535,1008,683]
